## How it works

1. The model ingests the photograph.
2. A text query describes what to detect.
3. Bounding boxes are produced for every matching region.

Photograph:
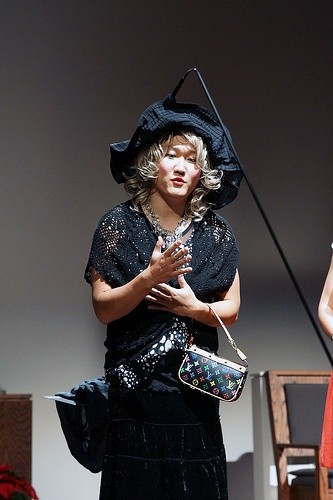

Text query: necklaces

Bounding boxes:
[145,195,192,249]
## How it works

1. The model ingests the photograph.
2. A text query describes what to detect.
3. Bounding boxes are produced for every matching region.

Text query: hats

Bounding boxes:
[109,100,243,210]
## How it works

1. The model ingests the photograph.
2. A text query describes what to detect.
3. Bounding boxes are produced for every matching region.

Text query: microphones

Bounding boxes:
[162,69,193,108]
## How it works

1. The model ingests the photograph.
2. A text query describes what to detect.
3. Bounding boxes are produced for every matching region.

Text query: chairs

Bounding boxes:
[264,369,333,500]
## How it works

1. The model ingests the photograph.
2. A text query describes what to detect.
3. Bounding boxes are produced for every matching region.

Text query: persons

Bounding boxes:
[84,100,244,500]
[318,244,333,469]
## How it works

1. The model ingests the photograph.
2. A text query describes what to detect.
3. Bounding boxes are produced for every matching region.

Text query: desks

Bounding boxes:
[0,393,33,500]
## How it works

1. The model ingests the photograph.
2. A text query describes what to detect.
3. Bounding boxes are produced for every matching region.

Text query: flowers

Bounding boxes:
[0,465,39,500]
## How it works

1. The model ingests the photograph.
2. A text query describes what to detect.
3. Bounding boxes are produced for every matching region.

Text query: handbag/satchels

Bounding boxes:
[41,316,189,473]
[178,303,249,402]
